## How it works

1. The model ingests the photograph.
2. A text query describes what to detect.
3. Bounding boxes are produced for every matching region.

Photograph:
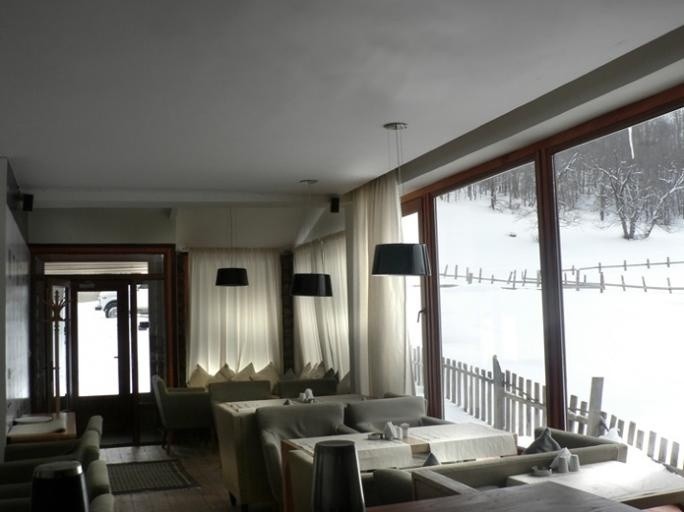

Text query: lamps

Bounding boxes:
[215,201,250,289]
[292,180,334,300]
[370,110,440,282]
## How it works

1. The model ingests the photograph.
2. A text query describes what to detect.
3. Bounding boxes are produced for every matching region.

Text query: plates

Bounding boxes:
[11,414,52,423]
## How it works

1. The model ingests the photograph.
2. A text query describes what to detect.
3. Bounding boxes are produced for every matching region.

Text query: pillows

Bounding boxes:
[186,362,329,389]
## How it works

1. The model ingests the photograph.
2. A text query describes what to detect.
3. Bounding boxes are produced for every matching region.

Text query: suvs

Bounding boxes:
[94,285,149,319]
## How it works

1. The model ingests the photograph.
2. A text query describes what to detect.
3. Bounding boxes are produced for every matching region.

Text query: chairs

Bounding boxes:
[254,395,683,512]
[1,405,115,510]
[151,371,339,445]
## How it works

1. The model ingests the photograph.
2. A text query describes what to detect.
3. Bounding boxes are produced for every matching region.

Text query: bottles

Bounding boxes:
[299,388,313,402]
[400,423,410,437]
[557,454,580,473]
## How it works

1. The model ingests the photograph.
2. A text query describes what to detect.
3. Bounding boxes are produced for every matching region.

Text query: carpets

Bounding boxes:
[107,459,198,497]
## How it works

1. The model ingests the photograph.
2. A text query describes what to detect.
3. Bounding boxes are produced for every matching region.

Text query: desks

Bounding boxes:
[208,395,373,511]
